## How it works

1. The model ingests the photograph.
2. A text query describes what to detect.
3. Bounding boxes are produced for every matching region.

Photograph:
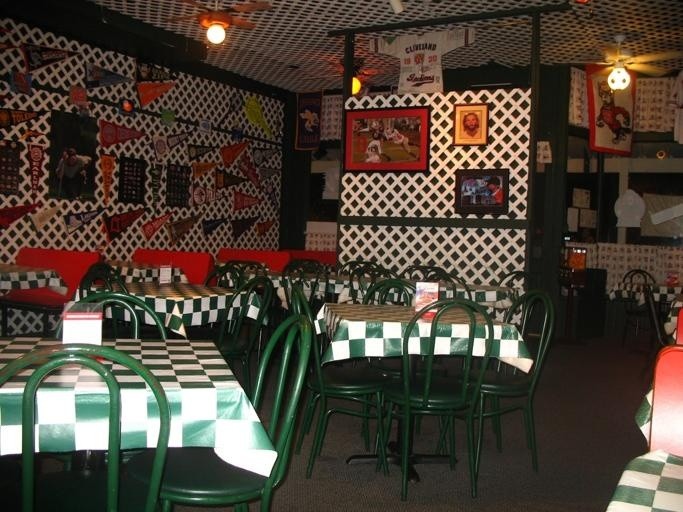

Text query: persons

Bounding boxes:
[416,288,438,313]
[461,113,481,139]
[461,175,503,207]
[55,146,92,197]
[353,116,421,163]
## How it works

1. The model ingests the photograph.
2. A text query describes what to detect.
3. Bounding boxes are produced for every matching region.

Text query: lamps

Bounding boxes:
[608,67,631,91]
[206,24,226,43]
[351,75,362,95]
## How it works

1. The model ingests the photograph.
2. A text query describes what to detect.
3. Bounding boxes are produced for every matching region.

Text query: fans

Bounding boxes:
[580,35,683,78]
[162,0,271,30]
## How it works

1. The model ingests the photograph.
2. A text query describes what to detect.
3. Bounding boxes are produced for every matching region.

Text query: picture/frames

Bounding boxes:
[453,103,489,146]
[343,106,431,173]
[454,168,509,214]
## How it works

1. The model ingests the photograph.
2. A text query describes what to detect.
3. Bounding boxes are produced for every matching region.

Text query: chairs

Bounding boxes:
[56,292,168,341]
[621,270,666,347]
[79,263,132,338]
[649,346,683,450]
[0,343,170,512]
[129,314,312,512]
[675,310,683,346]
